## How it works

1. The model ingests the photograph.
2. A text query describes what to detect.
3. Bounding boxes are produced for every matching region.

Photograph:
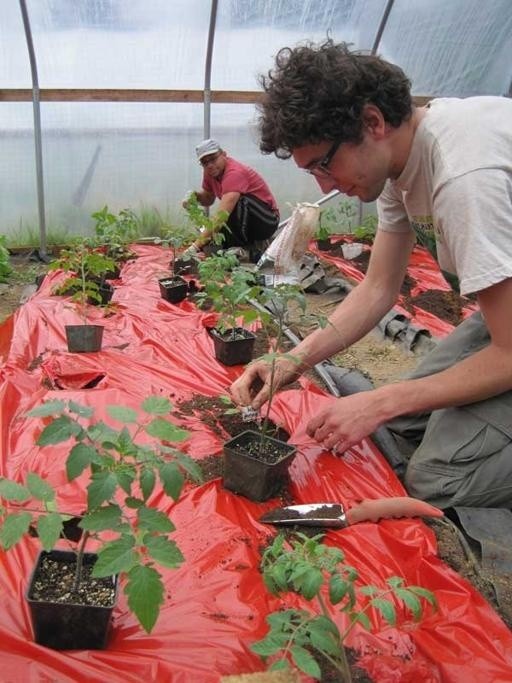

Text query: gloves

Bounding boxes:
[181,243,201,261]
[181,189,199,210]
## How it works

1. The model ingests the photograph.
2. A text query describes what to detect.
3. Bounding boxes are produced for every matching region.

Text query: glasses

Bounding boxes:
[305,139,343,179]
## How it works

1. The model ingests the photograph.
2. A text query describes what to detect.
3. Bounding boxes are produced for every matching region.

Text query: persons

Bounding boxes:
[230,41,512,521]
[180,139,280,262]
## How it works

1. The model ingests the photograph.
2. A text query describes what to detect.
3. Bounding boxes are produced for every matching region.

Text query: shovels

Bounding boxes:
[258,496,445,527]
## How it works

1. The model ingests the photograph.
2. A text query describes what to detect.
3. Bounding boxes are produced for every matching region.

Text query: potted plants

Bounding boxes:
[220,283,327,503]
[197,251,271,365]
[1,396,207,652]
[155,224,203,305]
[85,205,137,306]
[315,206,334,250]
[50,236,122,352]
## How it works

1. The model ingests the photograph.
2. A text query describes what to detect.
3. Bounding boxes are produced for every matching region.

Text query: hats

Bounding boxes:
[194,139,221,162]
